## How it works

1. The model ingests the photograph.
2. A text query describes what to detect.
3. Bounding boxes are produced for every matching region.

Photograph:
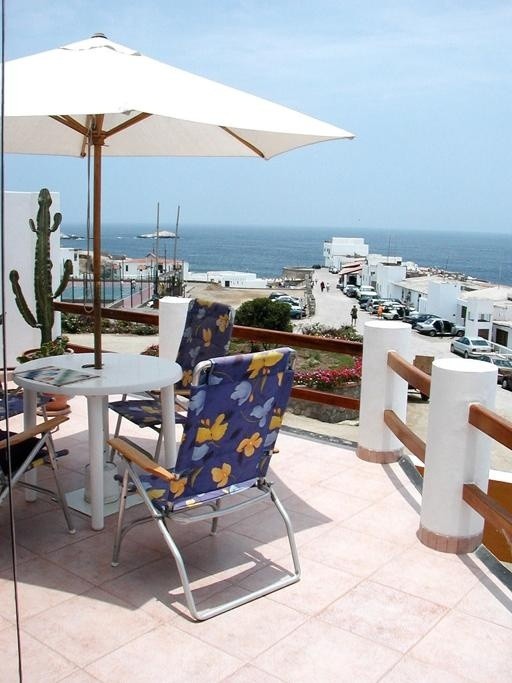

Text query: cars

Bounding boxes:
[337,282,418,319]
[450,335,512,388]
[403,313,465,337]
[270,292,306,319]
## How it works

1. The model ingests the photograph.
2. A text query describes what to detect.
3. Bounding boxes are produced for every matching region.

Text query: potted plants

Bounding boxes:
[8,186,78,416]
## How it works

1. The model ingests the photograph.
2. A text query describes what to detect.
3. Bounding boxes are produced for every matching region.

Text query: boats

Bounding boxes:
[138,230,178,238]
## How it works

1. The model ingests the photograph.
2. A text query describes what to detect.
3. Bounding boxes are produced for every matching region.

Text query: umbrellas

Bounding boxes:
[1,33,356,371]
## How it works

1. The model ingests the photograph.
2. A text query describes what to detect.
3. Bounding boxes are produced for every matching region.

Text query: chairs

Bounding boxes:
[0,348,78,550]
[108,346,301,622]
[109,300,238,464]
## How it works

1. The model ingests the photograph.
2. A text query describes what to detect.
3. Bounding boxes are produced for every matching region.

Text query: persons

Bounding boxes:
[131,278,136,289]
[367,300,374,317]
[377,302,384,320]
[320,281,324,292]
[350,305,358,327]
[326,282,331,292]
